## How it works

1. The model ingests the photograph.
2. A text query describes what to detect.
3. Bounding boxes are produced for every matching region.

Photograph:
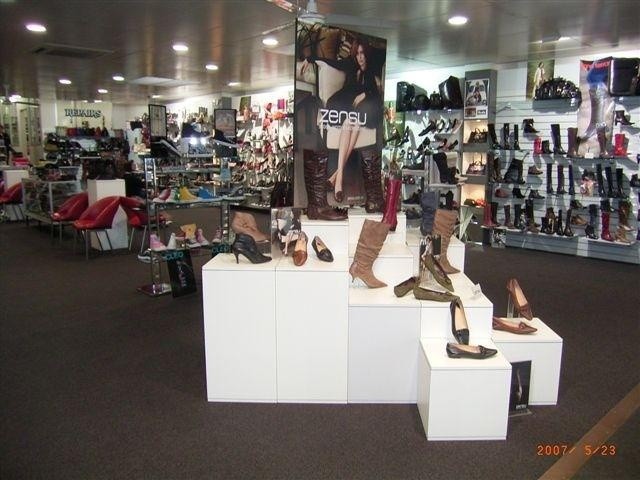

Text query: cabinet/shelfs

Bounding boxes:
[402,110,640,245]
[201,209,564,441]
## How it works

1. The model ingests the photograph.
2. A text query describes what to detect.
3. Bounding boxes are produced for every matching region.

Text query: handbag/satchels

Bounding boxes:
[395,75,464,113]
[466,159,485,176]
[467,127,489,144]
[533,76,578,100]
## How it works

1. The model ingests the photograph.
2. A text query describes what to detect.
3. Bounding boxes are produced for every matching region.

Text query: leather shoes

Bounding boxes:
[449,298,471,346]
[416,117,460,152]
[292,231,309,267]
[443,342,498,361]
[402,173,427,220]
[384,126,426,171]
[491,316,538,335]
[393,254,463,302]
[311,236,334,262]
[505,278,535,322]
[325,176,346,203]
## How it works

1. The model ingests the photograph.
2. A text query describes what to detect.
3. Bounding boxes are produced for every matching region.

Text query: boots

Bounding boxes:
[302,148,348,223]
[595,126,615,160]
[486,152,544,186]
[347,217,389,290]
[579,87,610,142]
[488,123,502,150]
[482,199,541,235]
[503,123,514,151]
[566,127,583,161]
[523,117,555,156]
[567,164,577,197]
[594,163,609,199]
[615,168,629,200]
[539,199,588,238]
[557,165,568,196]
[605,165,618,199]
[417,189,462,275]
[585,199,635,244]
[614,133,631,157]
[380,178,402,232]
[360,147,401,214]
[550,123,568,156]
[231,211,268,243]
[545,163,555,195]
[513,124,523,151]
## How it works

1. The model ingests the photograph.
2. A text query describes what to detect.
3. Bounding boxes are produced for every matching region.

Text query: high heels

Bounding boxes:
[493,187,545,201]
[231,233,272,265]
[614,109,637,126]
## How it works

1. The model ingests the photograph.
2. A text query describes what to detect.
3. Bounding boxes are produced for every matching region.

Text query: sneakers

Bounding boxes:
[152,185,246,204]
[147,226,225,253]
[179,122,208,140]
[231,103,294,208]
[211,128,240,148]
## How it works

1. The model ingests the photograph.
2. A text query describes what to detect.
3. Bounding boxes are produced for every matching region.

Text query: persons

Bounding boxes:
[531,61,546,98]
[299,37,377,203]
[473,81,481,103]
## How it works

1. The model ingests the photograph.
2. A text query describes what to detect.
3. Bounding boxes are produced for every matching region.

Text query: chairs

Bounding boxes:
[120,196,172,253]
[0,183,25,223]
[72,196,120,260]
[51,191,87,245]
